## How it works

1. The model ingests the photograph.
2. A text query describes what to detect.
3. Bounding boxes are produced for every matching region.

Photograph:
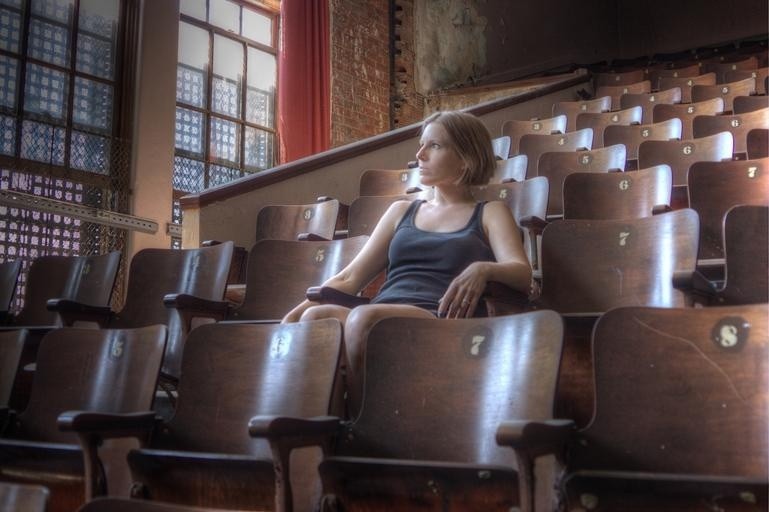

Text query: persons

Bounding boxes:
[279,110,533,417]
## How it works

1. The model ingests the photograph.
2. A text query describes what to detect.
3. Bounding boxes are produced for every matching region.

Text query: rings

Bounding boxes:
[462,299,470,304]
